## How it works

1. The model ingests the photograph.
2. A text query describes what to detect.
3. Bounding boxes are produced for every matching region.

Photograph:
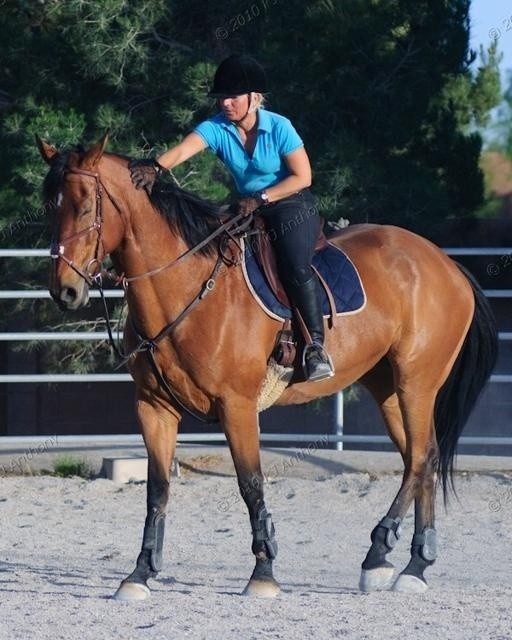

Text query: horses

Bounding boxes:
[34,127,500,602]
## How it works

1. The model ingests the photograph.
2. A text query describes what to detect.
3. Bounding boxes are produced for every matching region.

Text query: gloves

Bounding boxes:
[231,198,258,220]
[131,164,158,195]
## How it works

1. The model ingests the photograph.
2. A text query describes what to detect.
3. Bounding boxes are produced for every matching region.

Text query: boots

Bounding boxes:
[293,274,334,384]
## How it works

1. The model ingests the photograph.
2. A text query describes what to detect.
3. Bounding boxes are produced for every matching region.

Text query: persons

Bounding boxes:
[129,54,333,382]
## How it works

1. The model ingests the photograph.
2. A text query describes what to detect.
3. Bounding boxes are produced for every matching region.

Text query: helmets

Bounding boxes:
[206,54,269,98]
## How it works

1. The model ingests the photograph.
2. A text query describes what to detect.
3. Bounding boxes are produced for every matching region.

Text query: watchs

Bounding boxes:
[260,189,268,204]
